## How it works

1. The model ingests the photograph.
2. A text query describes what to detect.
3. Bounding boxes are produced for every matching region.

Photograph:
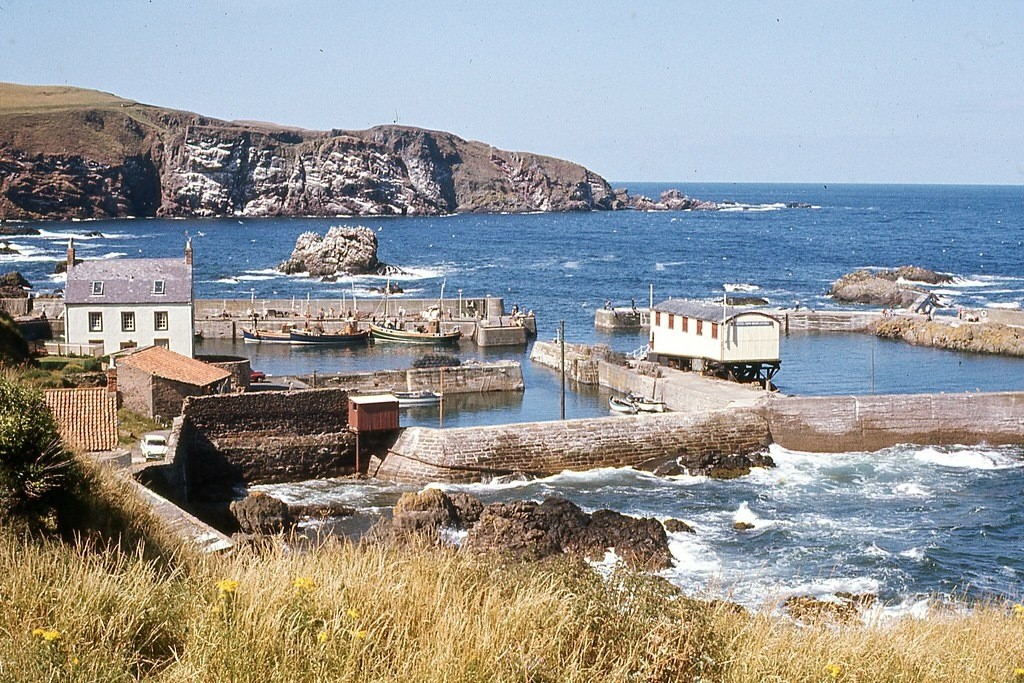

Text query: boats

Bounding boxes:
[396,392,446,408]
[369,271,463,345]
[290,329,370,347]
[242,326,292,345]
[610,395,668,415]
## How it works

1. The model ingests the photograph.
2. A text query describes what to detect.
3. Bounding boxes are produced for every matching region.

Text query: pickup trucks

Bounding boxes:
[141,435,168,460]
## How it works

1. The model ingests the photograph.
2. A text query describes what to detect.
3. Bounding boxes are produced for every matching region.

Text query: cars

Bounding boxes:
[250,368,267,384]
